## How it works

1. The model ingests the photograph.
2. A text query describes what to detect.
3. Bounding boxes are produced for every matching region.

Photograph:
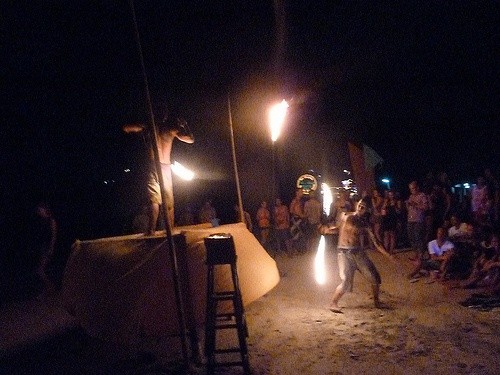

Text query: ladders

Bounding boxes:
[202,254,253,375]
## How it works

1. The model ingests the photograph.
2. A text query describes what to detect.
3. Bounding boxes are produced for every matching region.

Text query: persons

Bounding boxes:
[123,104,195,236]
[199,166,500,312]
[34,203,57,299]
